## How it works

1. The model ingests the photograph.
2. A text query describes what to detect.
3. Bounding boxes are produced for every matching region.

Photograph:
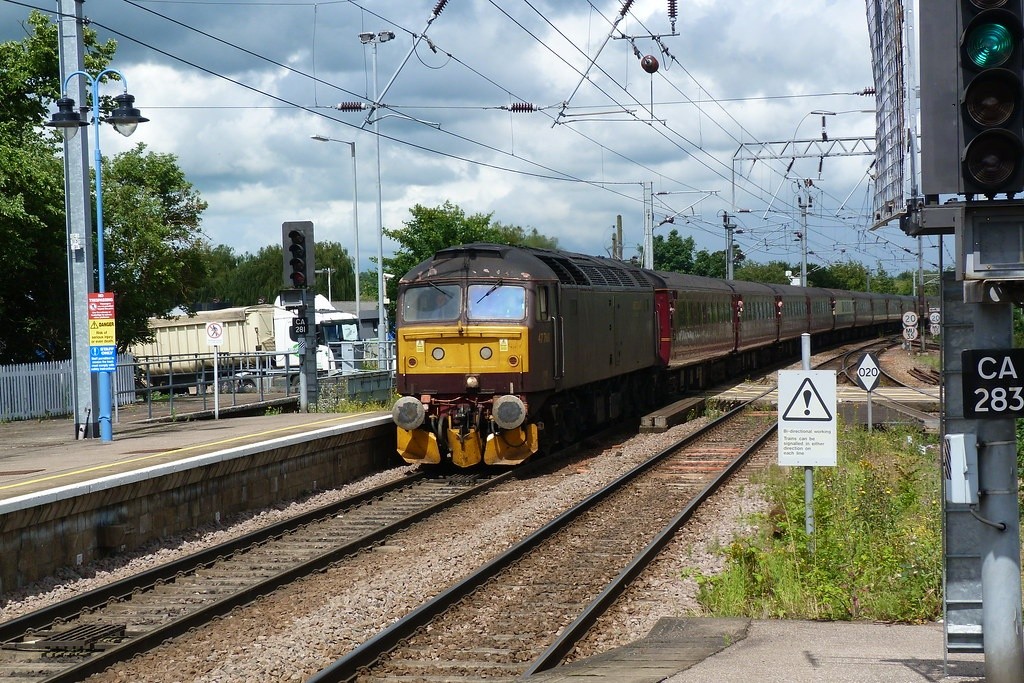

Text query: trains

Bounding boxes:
[392,242,939,470]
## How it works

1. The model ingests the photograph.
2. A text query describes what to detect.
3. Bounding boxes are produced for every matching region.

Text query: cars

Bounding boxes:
[234,362,299,393]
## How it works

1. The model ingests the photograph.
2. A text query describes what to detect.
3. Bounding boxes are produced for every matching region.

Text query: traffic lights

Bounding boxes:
[959,0,1024,195]
[282,221,317,287]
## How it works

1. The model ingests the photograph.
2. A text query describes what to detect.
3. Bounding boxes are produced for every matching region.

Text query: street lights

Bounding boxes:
[311,134,360,324]
[44,69,149,442]
[358,30,395,370]
[315,268,337,304]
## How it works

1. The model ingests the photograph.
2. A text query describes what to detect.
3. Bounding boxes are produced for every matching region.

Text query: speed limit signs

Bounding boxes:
[903,311,917,326]
[930,324,940,335]
[902,327,917,340]
[930,313,940,323]
